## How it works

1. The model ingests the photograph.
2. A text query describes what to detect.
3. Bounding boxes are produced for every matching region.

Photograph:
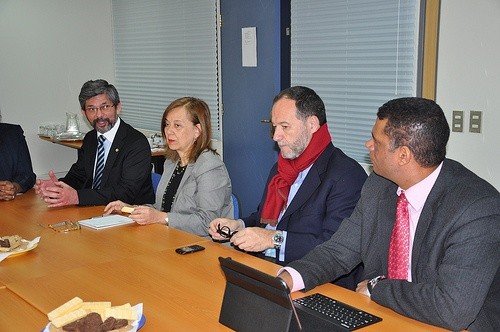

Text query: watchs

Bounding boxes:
[271,228,284,249]
[366,275,387,294]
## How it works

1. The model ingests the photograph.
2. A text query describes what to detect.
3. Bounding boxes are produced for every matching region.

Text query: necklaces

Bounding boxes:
[175,159,188,174]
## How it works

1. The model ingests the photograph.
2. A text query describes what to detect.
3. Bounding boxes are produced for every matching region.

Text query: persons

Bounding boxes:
[208,85,370,289]
[0,121,38,202]
[33,79,157,207]
[104,96,236,247]
[277,97,500,332]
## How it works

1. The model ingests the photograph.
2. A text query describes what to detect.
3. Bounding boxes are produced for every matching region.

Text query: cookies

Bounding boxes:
[121,206,135,212]
[47,296,139,332]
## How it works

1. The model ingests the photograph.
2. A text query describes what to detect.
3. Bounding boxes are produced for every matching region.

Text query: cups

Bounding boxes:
[38,124,65,138]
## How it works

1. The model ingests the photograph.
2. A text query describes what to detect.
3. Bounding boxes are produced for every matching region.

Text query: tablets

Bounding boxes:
[218,256,303,332]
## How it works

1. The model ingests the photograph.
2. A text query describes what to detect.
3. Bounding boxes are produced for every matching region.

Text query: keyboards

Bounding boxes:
[292,292,383,331]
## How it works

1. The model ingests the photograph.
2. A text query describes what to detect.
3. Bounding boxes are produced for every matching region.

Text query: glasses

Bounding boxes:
[84,102,116,113]
[216,224,238,239]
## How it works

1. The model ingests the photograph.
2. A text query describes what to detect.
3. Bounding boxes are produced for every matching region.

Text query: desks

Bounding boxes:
[0,187,456,332]
[39,133,169,155]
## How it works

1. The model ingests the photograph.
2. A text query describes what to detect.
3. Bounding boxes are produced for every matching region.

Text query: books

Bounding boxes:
[78,212,136,229]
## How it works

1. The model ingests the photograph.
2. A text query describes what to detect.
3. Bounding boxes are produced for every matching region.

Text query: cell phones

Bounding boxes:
[175,245,205,255]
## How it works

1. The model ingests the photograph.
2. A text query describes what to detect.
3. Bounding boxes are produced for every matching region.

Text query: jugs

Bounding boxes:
[65,112,80,135]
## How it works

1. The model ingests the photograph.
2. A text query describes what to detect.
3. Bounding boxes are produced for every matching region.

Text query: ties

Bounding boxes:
[388,190,410,282]
[93,135,107,190]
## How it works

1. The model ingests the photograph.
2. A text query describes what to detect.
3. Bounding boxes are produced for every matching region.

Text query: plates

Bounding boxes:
[0,240,38,259]
[41,310,146,332]
